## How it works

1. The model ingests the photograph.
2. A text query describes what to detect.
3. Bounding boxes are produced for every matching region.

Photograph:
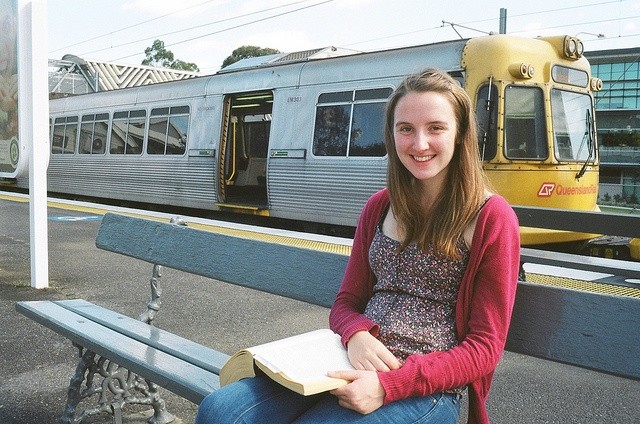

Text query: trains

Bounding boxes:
[0,36,602,249]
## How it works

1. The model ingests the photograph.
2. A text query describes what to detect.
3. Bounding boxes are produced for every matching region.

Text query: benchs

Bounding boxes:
[14,213,640,423]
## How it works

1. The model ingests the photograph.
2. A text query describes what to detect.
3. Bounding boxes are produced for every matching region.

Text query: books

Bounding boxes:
[218,329,356,397]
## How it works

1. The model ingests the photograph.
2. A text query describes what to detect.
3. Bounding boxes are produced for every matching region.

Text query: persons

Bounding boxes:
[194,66,522,424]
